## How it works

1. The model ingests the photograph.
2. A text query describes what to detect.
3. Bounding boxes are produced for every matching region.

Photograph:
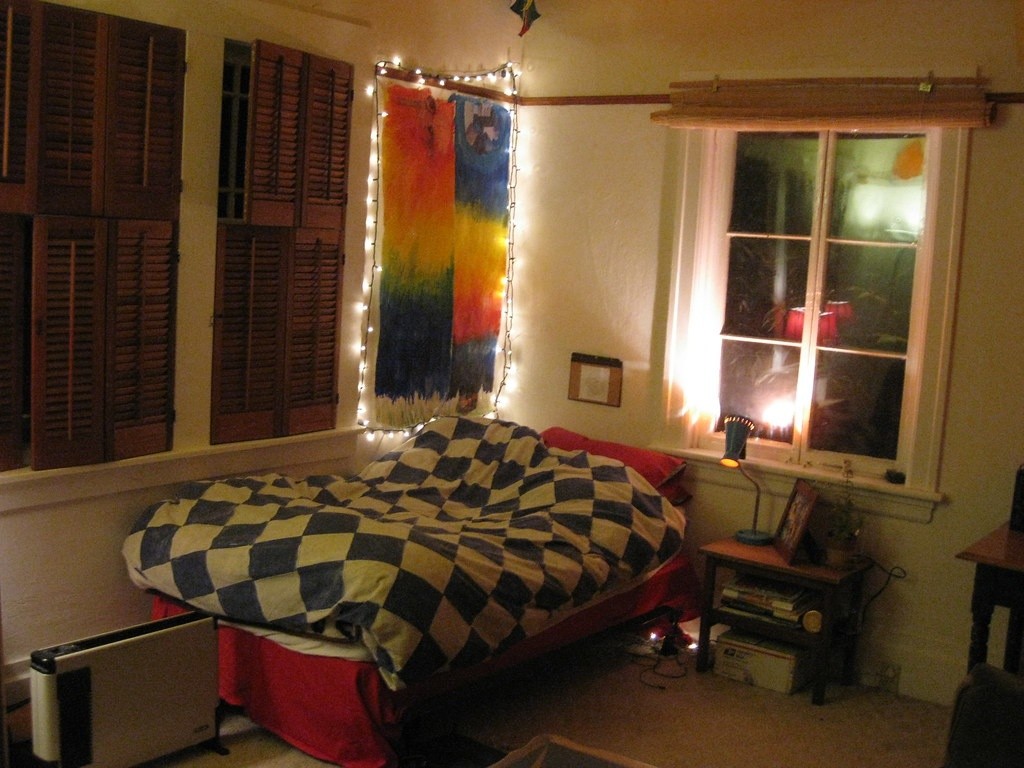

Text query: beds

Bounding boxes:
[149,436,694,768]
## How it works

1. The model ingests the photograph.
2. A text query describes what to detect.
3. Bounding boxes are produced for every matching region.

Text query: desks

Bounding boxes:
[697,537,875,706]
[956,521,1023,675]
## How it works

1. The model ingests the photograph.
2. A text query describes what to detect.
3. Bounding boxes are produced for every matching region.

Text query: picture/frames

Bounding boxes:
[772,480,818,566]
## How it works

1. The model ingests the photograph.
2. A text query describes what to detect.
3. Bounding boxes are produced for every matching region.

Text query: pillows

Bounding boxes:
[538,427,693,507]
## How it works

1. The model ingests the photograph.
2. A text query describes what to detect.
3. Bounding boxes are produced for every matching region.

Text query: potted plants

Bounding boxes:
[817,458,869,564]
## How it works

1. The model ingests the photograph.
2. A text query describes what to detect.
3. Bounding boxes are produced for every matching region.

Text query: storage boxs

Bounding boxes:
[712,628,810,695]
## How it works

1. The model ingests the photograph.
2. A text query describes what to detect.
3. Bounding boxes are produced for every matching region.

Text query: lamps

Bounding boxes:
[719,413,772,546]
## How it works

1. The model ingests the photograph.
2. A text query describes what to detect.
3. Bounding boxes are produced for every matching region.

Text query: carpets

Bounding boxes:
[486,731,657,768]
